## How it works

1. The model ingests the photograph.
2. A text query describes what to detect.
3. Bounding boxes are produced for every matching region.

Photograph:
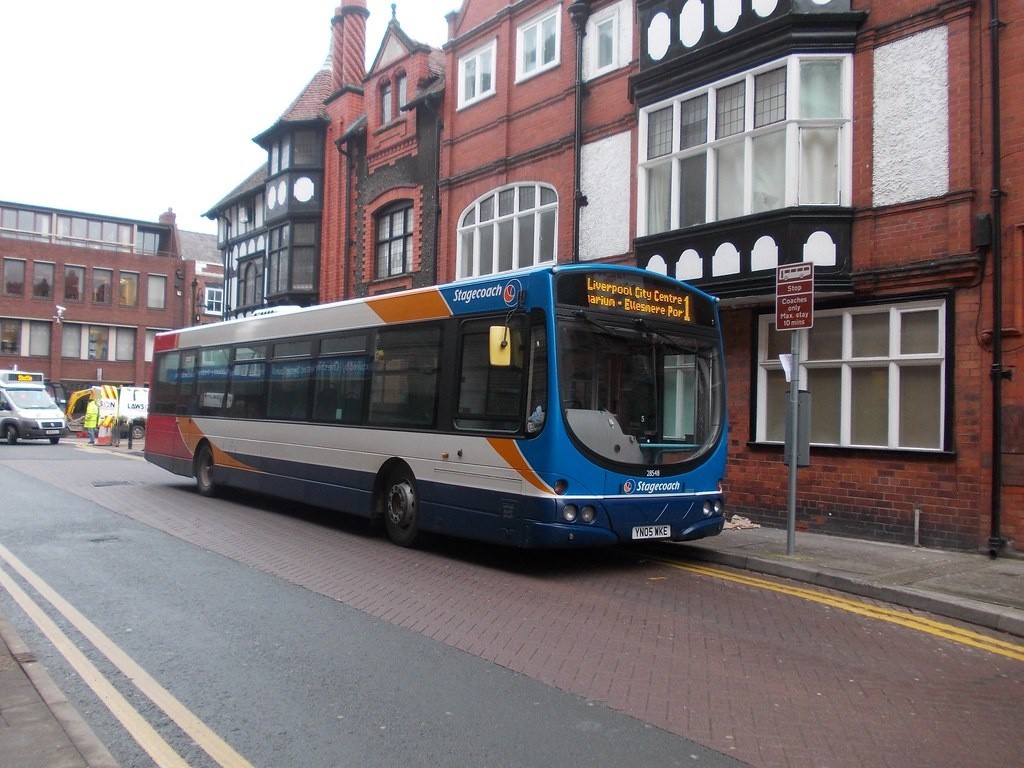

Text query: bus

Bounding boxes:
[143,264,728,551]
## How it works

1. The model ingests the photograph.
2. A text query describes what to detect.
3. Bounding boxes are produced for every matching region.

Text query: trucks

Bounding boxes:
[92,385,149,439]
[0,370,66,446]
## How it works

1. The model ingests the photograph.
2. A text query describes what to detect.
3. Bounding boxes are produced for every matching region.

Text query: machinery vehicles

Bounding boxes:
[66,389,92,431]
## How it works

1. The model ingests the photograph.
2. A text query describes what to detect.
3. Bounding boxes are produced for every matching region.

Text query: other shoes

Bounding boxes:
[89,441,94,444]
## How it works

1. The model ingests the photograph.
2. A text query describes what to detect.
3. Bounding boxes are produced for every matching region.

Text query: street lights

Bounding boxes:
[191,277,208,327]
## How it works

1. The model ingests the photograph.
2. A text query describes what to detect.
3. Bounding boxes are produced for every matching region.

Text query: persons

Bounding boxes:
[84,399,98,444]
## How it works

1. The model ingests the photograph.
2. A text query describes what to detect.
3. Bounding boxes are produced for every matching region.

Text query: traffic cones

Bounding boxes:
[94,423,112,446]
